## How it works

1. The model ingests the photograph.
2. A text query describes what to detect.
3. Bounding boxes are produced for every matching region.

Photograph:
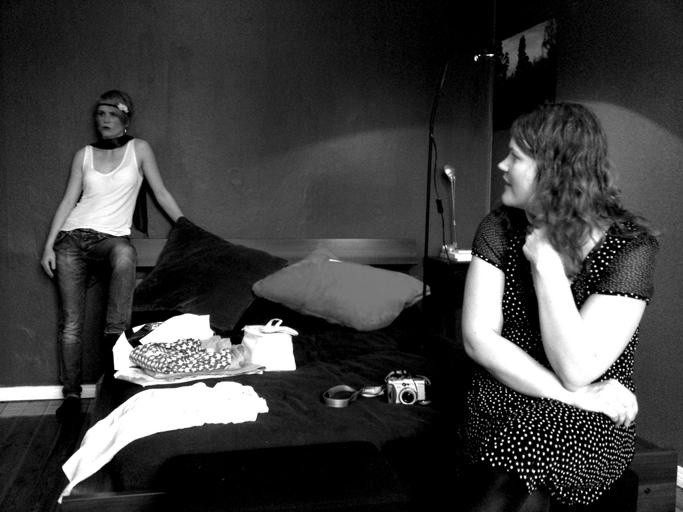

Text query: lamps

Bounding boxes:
[442,163,461,249]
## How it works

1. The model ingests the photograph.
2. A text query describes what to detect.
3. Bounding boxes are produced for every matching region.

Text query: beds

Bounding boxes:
[96,234,488,512]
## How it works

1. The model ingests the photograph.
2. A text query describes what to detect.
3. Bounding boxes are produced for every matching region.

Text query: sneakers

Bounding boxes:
[56,395,82,417]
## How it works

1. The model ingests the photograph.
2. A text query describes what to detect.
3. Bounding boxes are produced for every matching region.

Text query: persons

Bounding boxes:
[40,89,183,416]
[461,103,658,510]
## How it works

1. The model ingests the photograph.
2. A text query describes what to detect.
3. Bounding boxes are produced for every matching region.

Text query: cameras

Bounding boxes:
[386,376,426,405]
